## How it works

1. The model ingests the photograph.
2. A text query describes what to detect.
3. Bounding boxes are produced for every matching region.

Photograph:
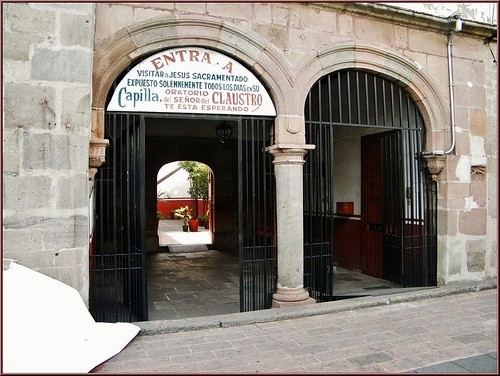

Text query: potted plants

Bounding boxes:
[180,206,192,232]
[203,210,210,229]
